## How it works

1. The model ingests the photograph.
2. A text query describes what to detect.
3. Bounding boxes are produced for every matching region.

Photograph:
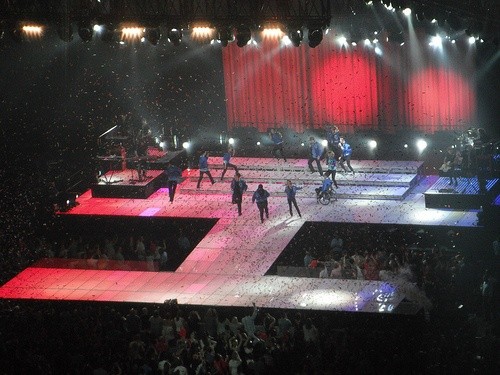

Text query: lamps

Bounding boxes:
[7,1,500,47]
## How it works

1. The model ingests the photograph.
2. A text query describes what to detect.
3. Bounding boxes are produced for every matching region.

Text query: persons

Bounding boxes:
[328,126,341,162]
[132,142,154,182]
[196,150,216,191]
[338,136,355,175]
[165,160,182,204]
[269,128,287,162]
[308,137,323,177]
[252,184,270,223]
[231,173,248,216]
[317,173,333,199]
[285,180,301,218]
[0,214,499,375]
[445,147,458,187]
[324,151,339,189]
[220,150,238,181]
